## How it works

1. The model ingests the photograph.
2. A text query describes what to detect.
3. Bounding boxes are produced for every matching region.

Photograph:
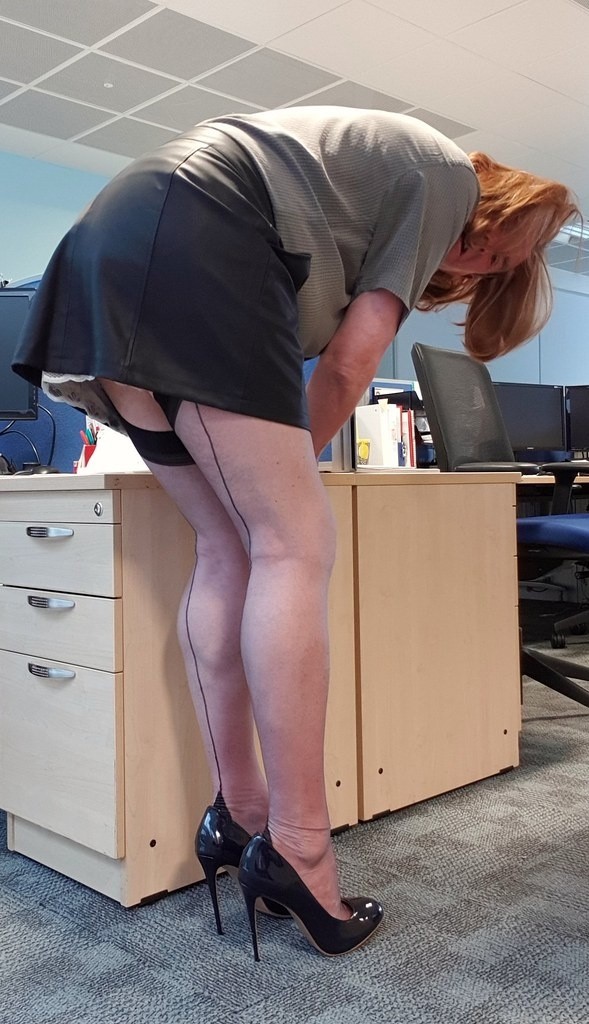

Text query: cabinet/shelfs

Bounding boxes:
[0,470,357,909]
[354,472,523,822]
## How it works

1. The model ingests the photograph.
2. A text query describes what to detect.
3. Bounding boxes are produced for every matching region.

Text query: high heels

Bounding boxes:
[195,805,294,937]
[238,833,383,964]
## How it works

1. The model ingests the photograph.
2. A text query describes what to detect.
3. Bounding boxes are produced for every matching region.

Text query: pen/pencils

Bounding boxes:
[79,422,100,446]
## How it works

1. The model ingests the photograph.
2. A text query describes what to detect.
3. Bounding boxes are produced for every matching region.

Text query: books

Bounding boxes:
[355,404,417,468]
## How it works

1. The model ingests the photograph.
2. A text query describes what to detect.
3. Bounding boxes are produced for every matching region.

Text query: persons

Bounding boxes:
[64,104,547,957]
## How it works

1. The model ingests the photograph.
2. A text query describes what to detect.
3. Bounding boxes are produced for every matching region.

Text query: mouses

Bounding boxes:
[30,464,59,474]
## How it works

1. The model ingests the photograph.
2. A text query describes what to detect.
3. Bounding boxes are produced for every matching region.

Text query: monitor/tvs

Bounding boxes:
[0,288,37,420]
[565,385,588,452]
[491,382,566,451]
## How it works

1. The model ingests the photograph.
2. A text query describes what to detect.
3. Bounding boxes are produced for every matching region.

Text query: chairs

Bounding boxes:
[411,341,589,650]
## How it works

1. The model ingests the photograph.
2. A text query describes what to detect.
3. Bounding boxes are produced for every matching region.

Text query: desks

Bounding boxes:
[517,474,589,483]
